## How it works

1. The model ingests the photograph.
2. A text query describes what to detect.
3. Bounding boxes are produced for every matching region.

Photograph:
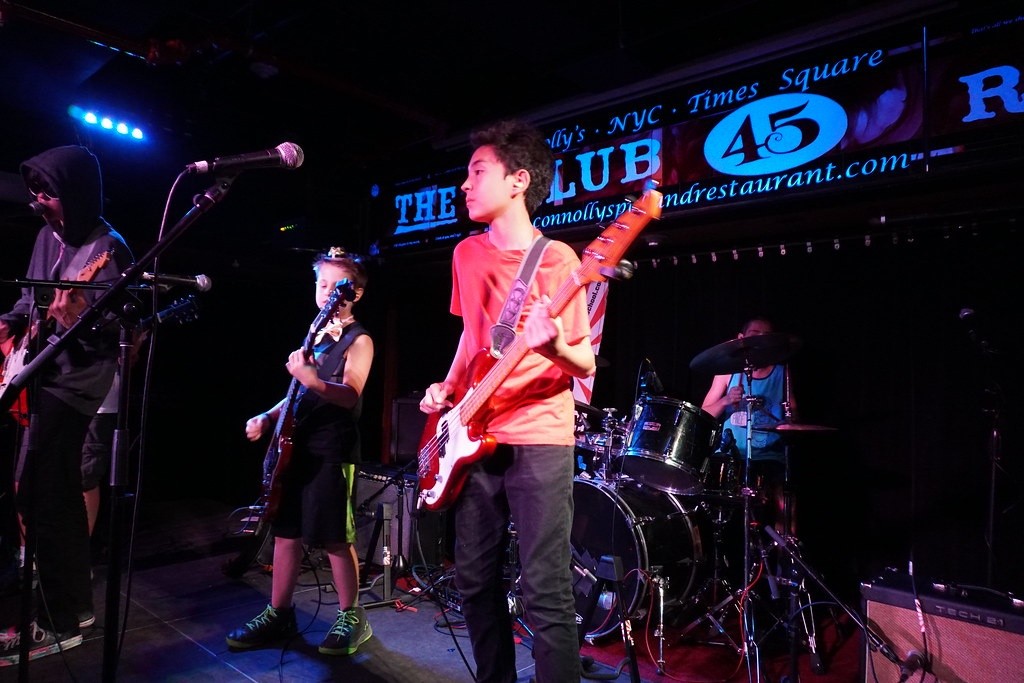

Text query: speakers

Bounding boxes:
[861,568,1024,683]
[390,398,427,474]
[350,466,430,574]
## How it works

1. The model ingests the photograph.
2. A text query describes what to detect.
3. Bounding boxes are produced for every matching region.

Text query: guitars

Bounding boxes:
[413,191,664,513]
[0,293,203,427]
[260,282,356,526]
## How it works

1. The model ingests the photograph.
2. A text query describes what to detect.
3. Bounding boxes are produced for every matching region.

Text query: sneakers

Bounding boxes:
[225,601,297,648]
[318,605,373,655]
[0,616,82,667]
[78,608,96,629]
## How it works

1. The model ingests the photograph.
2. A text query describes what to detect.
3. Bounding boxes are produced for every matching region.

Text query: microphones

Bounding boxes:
[356,509,378,520]
[899,650,927,683]
[649,362,664,393]
[960,308,977,341]
[141,272,212,291]
[0,202,48,221]
[187,141,304,176]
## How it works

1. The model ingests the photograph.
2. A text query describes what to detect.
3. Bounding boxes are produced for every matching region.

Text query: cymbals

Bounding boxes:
[574,400,604,415]
[740,422,839,433]
[690,331,807,373]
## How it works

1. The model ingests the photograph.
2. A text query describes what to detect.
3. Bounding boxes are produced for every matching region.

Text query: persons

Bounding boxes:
[14,371,119,536]
[418,121,595,683]
[701,314,796,549]
[0,144,136,666]
[225,246,373,655]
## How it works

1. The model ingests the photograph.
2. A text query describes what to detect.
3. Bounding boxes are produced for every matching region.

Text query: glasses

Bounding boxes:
[27,180,61,201]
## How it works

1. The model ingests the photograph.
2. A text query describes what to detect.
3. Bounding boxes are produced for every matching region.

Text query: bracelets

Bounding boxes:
[263,412,272,427]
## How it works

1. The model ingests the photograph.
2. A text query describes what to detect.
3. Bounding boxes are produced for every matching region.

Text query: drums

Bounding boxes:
[571,475,697,640]
[615,393,723,497]
[573,429,627,481]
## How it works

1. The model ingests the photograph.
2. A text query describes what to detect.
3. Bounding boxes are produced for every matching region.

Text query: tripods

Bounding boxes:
[357,458,419,596]
[666,356,845,683]
